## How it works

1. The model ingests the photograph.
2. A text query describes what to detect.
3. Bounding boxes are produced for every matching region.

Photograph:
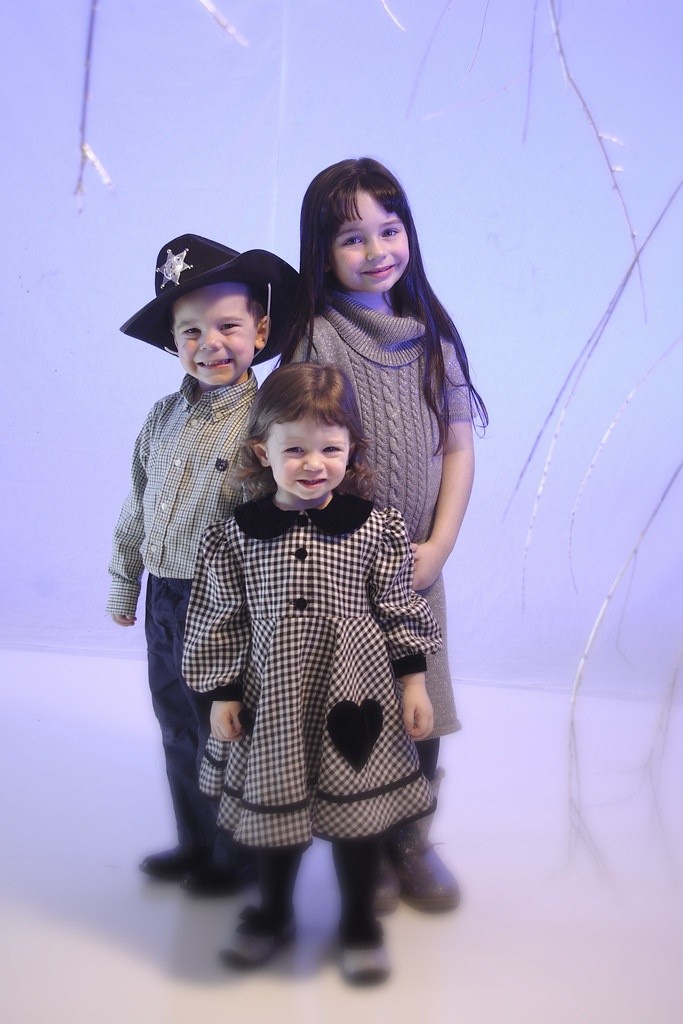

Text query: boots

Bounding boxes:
[354,767,460,910]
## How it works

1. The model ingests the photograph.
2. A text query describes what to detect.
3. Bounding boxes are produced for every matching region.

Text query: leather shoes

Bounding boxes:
[140,844,214,876]
[181,862,255,893]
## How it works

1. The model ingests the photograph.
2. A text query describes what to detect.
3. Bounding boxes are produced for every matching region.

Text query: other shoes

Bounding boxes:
[337,918,390,978]
[218,906,296,965]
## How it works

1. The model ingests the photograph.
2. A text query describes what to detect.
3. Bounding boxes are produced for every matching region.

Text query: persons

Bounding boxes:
[102,234,310,900]
[277,154,491,916]
[179,362,434,986]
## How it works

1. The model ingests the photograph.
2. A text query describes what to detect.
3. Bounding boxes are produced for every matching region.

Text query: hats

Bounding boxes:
[119,234,299,367]
